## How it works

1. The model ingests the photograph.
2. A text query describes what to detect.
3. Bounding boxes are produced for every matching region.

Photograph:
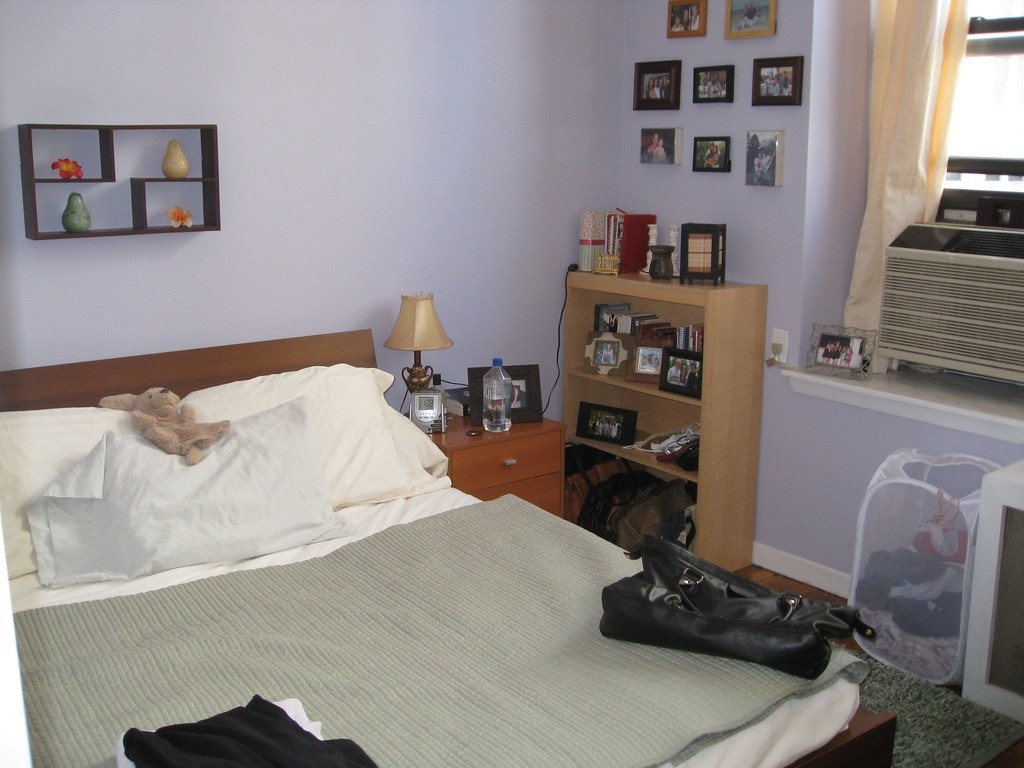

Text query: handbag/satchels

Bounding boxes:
[599,529,876,682]
[562,418,701,553]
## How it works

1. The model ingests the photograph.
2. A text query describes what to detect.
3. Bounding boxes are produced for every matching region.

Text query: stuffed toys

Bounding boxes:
[99,386,231,466]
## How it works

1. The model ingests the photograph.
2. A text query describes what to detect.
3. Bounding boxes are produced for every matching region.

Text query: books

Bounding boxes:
[444,388,470,417]
[594,302,703,354]
[577,209,657,274]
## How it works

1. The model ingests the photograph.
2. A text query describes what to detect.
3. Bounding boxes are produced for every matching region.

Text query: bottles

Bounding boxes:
[648,245,676,280]
[482,358,512,432]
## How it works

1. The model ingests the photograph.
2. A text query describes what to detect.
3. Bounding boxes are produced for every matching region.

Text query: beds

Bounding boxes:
[0,328,897,768]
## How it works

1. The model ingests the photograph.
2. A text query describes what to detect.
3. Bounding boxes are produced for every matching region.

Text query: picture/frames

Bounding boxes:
[666,0,708,39]
[658,346,703,401]
[804,324,879,383]
[576,401,638,447]
[627,336,675,384]
[632,60,683,111]
[693,135,730,173]
[693,65,735,103]
[466,363,544,427]
[582,329,631,376]
[751,56,804,107]
[724,0,777,40]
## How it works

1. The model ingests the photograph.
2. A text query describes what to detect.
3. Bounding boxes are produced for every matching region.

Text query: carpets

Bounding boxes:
[813,629,1024,768]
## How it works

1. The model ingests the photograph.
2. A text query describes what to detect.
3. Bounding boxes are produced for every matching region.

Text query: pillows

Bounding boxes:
[27,394,355,588]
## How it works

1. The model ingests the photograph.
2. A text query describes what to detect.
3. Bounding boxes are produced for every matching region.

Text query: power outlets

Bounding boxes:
[772,328,789,364]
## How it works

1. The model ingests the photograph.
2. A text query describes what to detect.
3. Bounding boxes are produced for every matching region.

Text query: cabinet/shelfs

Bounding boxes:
[17,123,221,242]
[562,268,769,573]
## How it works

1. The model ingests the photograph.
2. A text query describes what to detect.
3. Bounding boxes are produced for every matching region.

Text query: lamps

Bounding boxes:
[384,292,454,395]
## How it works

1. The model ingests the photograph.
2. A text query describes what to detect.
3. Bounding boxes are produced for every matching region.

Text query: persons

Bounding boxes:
[823,339,854,367]
[671,4,700,32]
[707,73,723,98]
[754,148,775,186]
[765,70,791,97]
[510,382,526,408]
[704,141,720,168]
[667,359,699,391]
[601,343,615,365]
[592,413,621,438]
[648,133,669,162]
[646,75,670,100]
[738,2,765,31]
